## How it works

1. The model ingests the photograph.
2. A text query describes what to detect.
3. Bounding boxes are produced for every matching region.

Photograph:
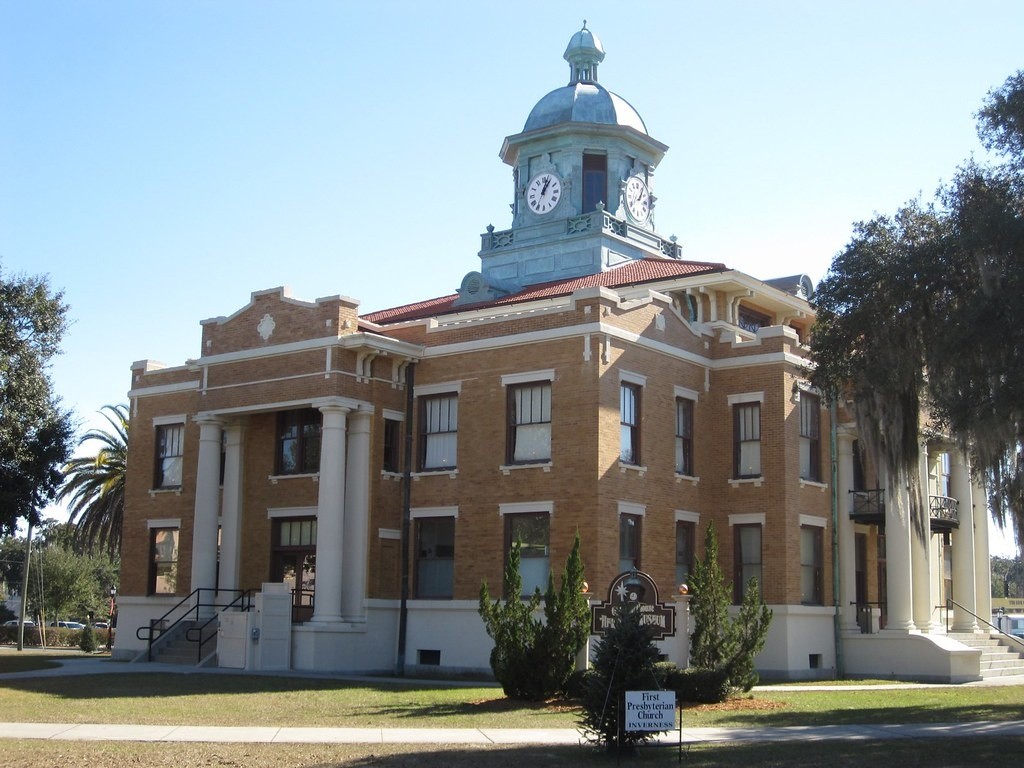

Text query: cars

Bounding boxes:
[50,621,84,631]
[92,621,108,628]
[3,620,35,628]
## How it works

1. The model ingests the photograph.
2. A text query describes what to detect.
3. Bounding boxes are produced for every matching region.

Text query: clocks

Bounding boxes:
[624,177,651,221]
[527,172,559,215]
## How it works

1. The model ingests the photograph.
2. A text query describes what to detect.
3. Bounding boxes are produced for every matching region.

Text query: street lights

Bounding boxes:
[105,583,117,651]
[996,606,1004,629]
